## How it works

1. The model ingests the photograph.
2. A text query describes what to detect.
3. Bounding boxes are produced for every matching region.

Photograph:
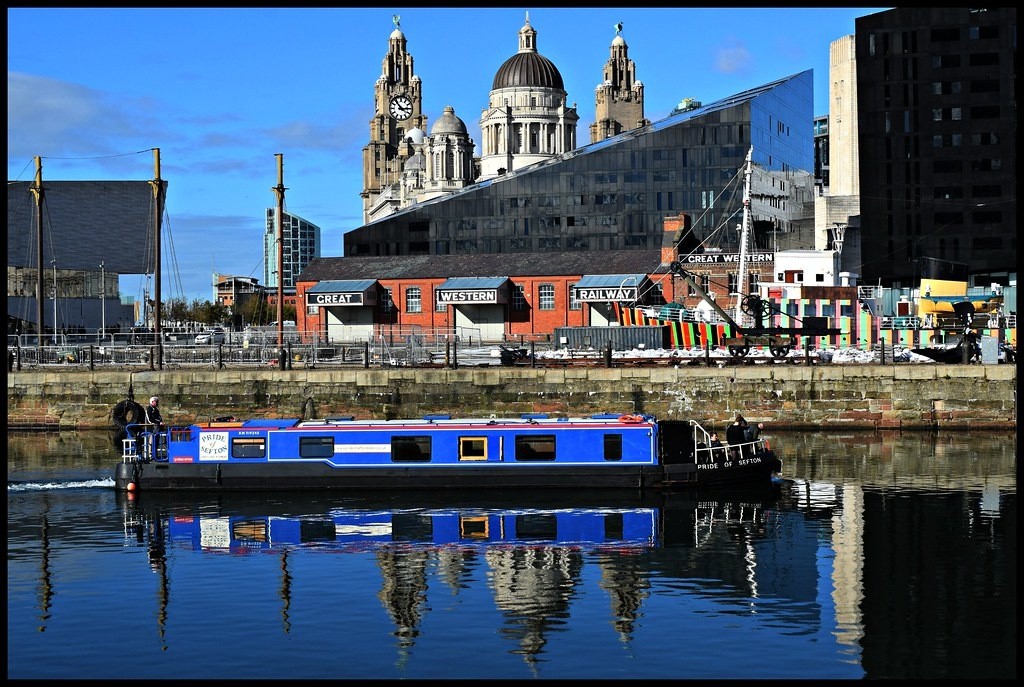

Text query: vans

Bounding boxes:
[268,320,296,327]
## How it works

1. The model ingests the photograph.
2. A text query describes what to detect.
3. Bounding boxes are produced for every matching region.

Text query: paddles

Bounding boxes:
[618,415,643,423]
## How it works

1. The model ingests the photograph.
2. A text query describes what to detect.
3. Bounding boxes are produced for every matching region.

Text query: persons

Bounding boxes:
[145,397,164,461]
[126,327,133,346]
[705,412,764,463]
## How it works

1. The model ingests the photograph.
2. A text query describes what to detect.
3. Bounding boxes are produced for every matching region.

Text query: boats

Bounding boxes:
[107,361,782,496]
[124,499,712,554]
[576,137,804,350]
[919,293,1005,313]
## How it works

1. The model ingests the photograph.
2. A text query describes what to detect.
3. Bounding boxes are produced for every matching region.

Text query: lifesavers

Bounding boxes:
[112,428,138,453]
[112,401,140,428]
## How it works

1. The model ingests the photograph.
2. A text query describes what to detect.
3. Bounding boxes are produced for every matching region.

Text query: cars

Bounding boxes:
[56,345,79,363]
[194,329,226,344]
